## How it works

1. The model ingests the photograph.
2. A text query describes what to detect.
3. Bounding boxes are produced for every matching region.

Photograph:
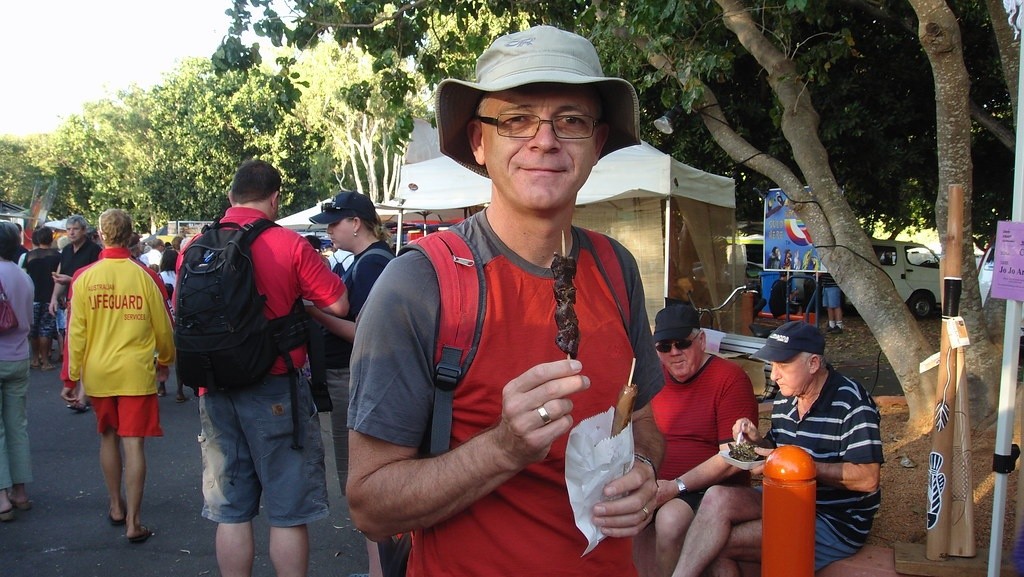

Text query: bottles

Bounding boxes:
[795,288,799,302]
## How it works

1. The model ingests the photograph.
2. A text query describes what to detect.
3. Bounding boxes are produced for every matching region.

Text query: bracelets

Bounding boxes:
[635,451,659,488]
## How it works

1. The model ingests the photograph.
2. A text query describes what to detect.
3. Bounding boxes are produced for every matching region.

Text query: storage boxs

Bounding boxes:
[719,450,766,470]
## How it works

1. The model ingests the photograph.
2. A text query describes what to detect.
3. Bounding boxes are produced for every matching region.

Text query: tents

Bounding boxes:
[273,194,472,259]
[398,136,749,335]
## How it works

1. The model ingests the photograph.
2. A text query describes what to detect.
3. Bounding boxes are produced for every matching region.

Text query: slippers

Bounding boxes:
[0,510,15,523]
[6,492,31,512]
[29,361,38,369]
[108,504,126,526]
[128,524,151,542]
[41,364,55,372]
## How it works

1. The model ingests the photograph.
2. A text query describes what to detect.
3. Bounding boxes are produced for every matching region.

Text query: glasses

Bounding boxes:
[472,113,602,139]
[655,330,701,353]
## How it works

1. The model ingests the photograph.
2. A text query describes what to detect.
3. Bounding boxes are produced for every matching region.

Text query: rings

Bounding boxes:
[536,406,551,424]
[642,506,649,522]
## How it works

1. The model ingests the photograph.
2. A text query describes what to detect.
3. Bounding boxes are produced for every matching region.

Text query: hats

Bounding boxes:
[433,25,642,181]
[653,304,700,343]
[747,320,825,363]
[309,191,375,226]
[304,235,323,253]
[128,232,143,250]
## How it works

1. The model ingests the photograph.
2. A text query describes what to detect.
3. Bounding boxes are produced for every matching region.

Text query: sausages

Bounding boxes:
[610,385,638,438]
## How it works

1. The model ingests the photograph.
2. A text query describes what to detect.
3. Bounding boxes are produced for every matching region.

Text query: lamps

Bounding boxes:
[654,103,690,135]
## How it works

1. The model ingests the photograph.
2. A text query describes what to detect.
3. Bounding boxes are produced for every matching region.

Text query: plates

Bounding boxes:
[720,451,766,470]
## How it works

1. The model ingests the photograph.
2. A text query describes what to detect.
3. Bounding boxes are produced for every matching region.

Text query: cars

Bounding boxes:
[692,261,763,287]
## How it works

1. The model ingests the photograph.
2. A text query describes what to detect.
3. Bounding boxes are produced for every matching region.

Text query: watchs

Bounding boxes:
[674,477,688,496]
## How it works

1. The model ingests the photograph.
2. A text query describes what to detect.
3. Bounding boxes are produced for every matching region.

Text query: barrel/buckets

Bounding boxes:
[803,313,815,325]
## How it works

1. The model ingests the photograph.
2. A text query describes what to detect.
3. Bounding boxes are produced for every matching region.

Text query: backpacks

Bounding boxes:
[174,215,334,451]
[332,253,354,279]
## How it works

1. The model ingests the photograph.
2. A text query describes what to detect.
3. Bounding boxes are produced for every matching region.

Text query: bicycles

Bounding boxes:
[687,284,778,339]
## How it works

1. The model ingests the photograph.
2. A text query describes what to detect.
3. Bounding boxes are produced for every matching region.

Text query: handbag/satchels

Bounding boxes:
[0,281,18,332]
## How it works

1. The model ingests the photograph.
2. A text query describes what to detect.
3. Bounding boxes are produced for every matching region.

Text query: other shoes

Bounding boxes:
[824,326,835,332]
[831,325,843,334]
[66,399,87,413]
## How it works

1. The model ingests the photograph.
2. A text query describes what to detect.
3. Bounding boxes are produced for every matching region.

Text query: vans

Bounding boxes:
[845,240,943,320]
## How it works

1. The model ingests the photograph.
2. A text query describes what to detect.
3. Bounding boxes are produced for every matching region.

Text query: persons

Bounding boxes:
[671,319,886,577]
[636,301,760,577]
[0,194,410,522]
[344,24,666,577]
[172,159,349,577]
[305,189,413,577]
[768,261,844,334]
[59,208,177,542]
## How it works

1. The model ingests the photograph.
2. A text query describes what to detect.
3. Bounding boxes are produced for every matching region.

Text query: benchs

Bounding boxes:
[737,545,906,577]
[720,332,779,402]
[759,312,803,322]
